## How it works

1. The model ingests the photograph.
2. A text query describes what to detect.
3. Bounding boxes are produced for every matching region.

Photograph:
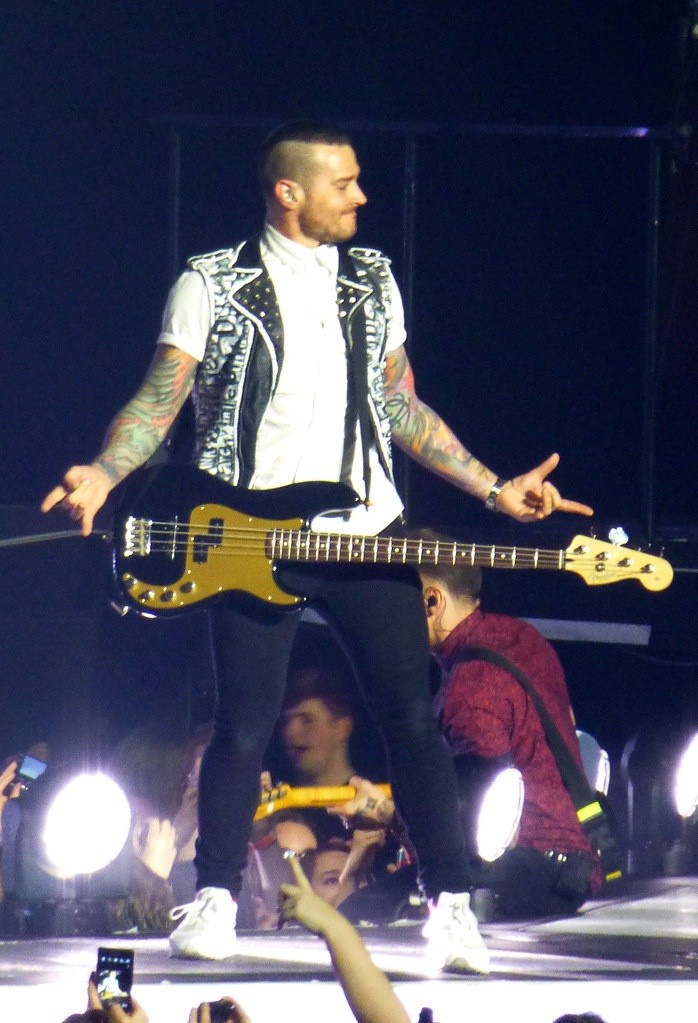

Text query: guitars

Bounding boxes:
[252,780,391,820]
[105,463,675,621]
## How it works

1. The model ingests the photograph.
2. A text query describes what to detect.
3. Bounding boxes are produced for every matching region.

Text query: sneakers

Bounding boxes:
[168,887,238,961]
[421,889,489,972]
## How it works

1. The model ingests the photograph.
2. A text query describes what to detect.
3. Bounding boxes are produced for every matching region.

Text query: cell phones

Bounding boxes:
[95,947,136,1013]
[197,1000,234,1023]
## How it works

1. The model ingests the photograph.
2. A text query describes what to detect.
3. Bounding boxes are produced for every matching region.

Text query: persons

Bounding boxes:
[98,970,129,999]
[0,529,610,1023]
[40,122,593,974]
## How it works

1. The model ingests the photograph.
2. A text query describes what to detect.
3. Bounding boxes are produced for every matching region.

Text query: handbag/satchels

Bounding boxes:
[583,789,636,897]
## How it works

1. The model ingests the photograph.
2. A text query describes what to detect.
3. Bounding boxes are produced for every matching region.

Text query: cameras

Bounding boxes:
[9,752,47,792]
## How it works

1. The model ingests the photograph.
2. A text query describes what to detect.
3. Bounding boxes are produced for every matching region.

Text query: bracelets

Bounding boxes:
[486,476,506,514]
